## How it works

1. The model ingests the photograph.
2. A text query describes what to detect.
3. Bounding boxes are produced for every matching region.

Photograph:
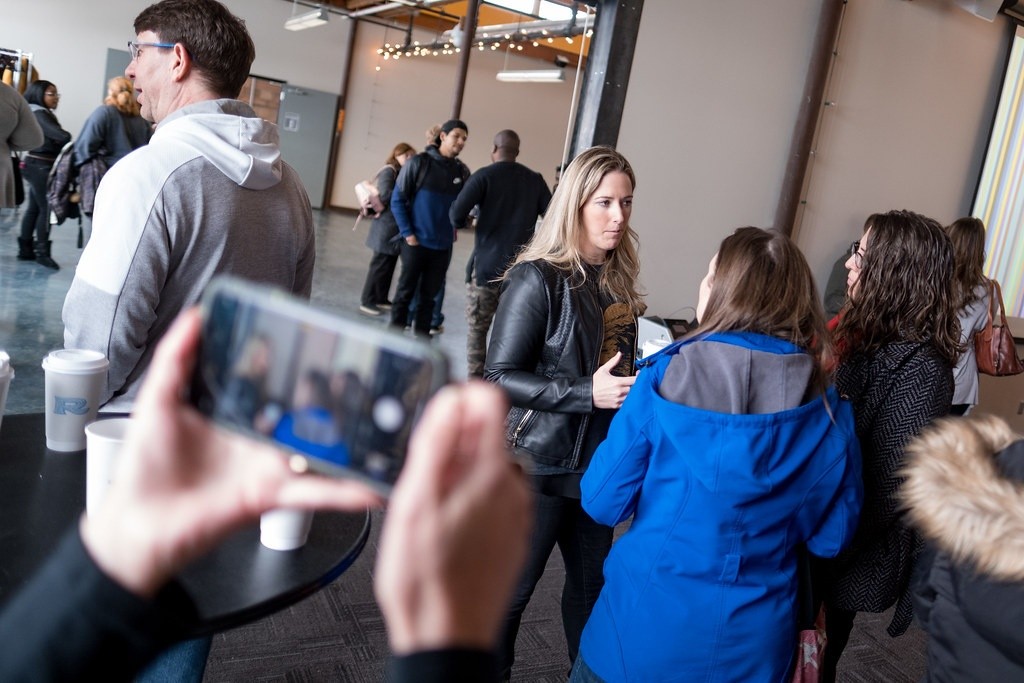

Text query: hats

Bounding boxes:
[434,119,470,148]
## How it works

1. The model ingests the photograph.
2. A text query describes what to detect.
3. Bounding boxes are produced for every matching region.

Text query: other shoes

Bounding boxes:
[359,303,382,317]
[375,299,392,312]
[427,326,445,338]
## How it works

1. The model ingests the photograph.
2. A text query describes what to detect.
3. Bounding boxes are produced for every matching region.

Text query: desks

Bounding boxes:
[0,411,373,646]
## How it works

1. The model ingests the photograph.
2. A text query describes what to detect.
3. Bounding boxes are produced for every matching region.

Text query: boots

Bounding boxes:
[33,239,61,271]
[17,236,37,261]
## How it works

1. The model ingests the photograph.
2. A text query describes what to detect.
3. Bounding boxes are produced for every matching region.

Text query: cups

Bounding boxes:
[41,348,111,453]
[260,506,314,551]
[83,416,135,525]
[0,351,15,428]
[642,339,671,358]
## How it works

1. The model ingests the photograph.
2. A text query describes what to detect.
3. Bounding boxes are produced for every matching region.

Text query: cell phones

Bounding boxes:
[177,275,448,500]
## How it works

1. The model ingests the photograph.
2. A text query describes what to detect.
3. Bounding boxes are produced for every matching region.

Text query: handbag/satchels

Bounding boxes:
[973,279,1024,377]
[351,165,397,232]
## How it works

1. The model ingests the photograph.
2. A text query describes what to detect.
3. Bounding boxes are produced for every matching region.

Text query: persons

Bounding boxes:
[569,226,865,683]
[17,80,71,270]
[62,1,316,412]
[387,120,471,340]
[482,146,648,683]
[783,210,969,683]
[824,214,880,320]
[73,76,157,168]
[897,412,1024,683]
[360,142,416,314]
[944,218,1001,417]
[0,80,44,209]
[215,332,363,467]
[0,305,535,683]
[448,130,562,382]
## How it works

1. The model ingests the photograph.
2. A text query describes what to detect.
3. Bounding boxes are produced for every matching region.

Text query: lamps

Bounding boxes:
[496,41,567,82]
[284,0,328,33]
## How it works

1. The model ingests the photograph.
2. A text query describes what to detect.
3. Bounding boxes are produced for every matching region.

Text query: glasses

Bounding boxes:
[42,91,61,98]
[850,240,865,270]
[127,40,177,60]
